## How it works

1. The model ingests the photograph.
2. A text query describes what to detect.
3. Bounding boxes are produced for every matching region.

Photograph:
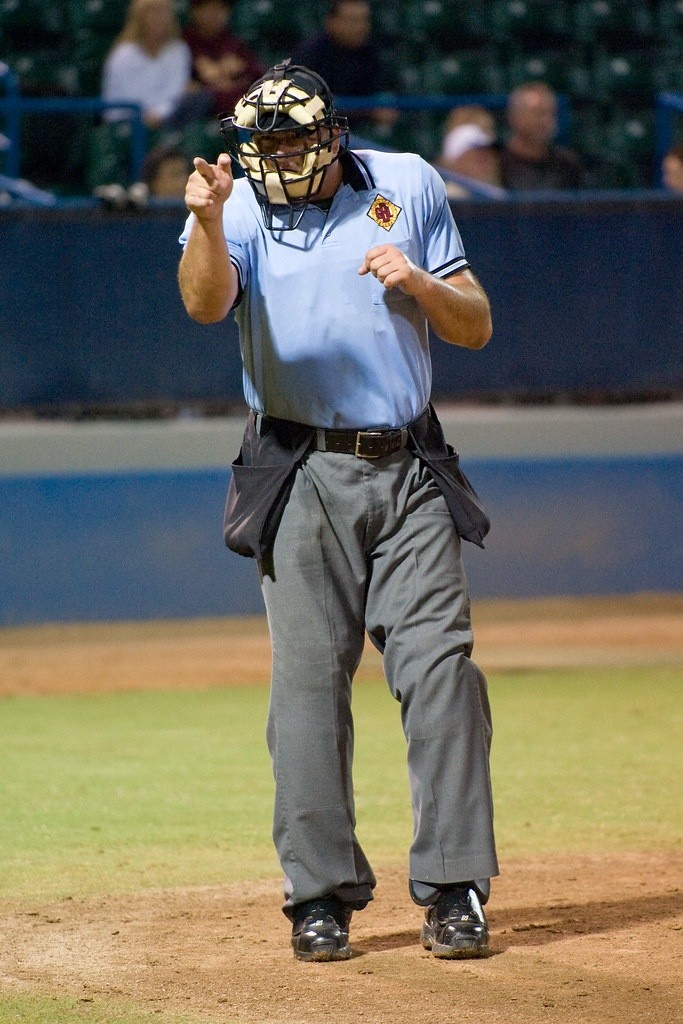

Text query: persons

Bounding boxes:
[178,62,501,963]
[99,0,586,207]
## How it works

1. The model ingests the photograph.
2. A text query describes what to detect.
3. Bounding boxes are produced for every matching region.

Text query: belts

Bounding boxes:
[313,427,409,459]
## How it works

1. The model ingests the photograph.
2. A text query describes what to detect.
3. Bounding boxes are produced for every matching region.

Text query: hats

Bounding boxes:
[443,125,495,163]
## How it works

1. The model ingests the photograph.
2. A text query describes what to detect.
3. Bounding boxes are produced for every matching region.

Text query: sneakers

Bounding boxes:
[292,897,352,961]
[422,888,490,957]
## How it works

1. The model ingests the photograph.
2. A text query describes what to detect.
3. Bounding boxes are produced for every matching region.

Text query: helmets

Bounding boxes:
[235,58,337,204]
